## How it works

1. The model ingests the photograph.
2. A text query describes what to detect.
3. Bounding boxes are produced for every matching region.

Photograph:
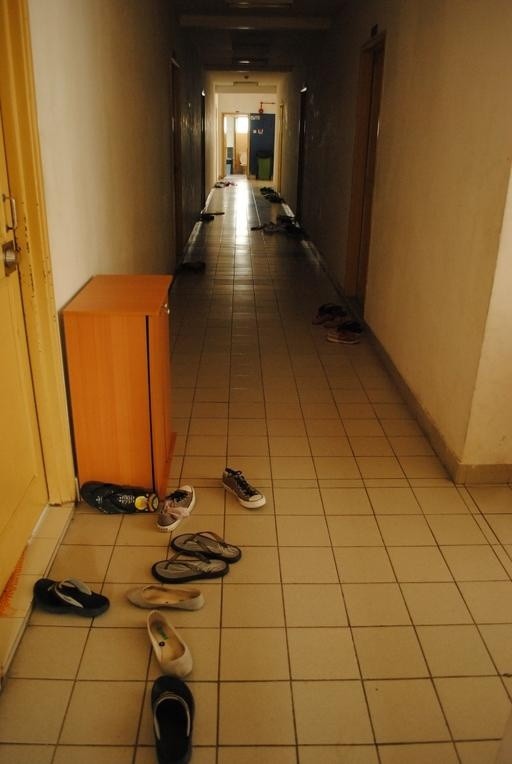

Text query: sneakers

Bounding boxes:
[222,468,266,508]
[157,484,195,530]
[127,585,203,764]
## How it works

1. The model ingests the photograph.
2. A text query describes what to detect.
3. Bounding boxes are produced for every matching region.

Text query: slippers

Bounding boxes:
[34,578,109,617]
[80,481,158,514]
[151,530,241,584]
[313,303,362,343]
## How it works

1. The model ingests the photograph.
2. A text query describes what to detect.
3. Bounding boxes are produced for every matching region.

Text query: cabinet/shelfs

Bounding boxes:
[61,272,176,500]
